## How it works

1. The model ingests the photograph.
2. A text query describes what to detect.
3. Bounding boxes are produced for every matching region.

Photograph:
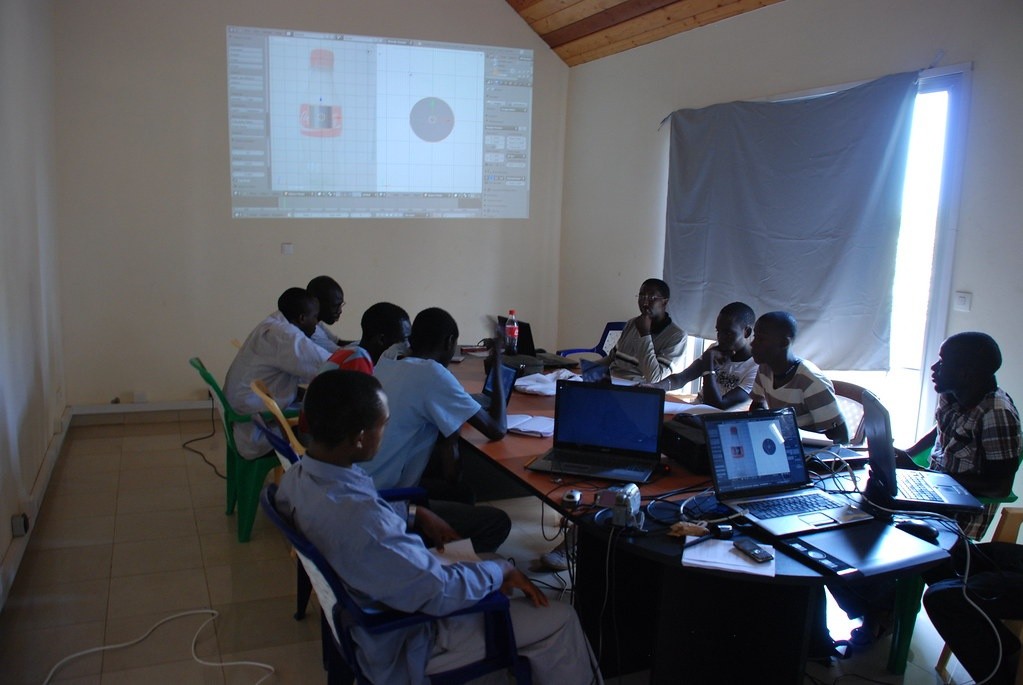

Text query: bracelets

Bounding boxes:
[702,370,716,376]
[665,378,672,391]
[408,504,416,532]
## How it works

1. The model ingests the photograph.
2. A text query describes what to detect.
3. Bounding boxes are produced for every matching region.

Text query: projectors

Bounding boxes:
[660,411,711,475]
[482,354,544,380]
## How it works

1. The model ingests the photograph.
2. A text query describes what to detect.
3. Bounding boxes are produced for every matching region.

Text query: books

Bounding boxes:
[682,534,777,578]
[506,414,554,437]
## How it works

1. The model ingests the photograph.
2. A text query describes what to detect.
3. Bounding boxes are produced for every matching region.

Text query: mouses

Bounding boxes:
[894,519,940,539]
[563,490,583,504]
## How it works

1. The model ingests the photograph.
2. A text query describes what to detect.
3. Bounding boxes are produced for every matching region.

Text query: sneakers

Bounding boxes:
[540,540,577,572]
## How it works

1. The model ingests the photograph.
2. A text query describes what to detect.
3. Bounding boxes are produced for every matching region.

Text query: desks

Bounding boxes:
[445,345,960,684]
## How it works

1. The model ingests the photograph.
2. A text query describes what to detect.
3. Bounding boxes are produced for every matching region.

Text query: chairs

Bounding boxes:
[829,380,880,446]
[189,356,532,685]
[880,445,1023,685]
[554,322,627,357]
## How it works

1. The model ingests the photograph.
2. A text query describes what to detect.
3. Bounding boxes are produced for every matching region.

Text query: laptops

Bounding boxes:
[703,406,875,537]
[800,441,871,474]
[496,314,580,368]
[861,388,985,511]
[471,363,520,410]
[526,379,668,485]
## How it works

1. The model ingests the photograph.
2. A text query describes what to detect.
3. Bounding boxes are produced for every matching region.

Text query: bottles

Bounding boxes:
[504,310,519,356]
[298,48,342,192]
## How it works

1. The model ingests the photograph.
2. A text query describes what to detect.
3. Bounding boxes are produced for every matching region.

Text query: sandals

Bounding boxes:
[847,617,891,651]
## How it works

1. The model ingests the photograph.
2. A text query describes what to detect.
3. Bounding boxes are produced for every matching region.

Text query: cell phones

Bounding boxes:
[733,538,773,562]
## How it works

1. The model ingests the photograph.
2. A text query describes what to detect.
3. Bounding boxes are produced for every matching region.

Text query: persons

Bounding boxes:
[851,332,1023,645]
[298,301,413,448]
[591,278,687,383]
[274,368,604,685]
[221,274,359,461]
[354,307,512,555]
[632,302,759,412]
[748,312,849,444]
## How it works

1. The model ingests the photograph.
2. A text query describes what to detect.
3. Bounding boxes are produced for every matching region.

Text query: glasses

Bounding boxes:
[324,302,346,310]
[634,294,667,302]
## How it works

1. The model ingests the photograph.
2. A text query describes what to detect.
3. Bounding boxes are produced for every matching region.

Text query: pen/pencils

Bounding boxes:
[638,375,645,385]
[523,456,538,468]
[682,533,717,548]
